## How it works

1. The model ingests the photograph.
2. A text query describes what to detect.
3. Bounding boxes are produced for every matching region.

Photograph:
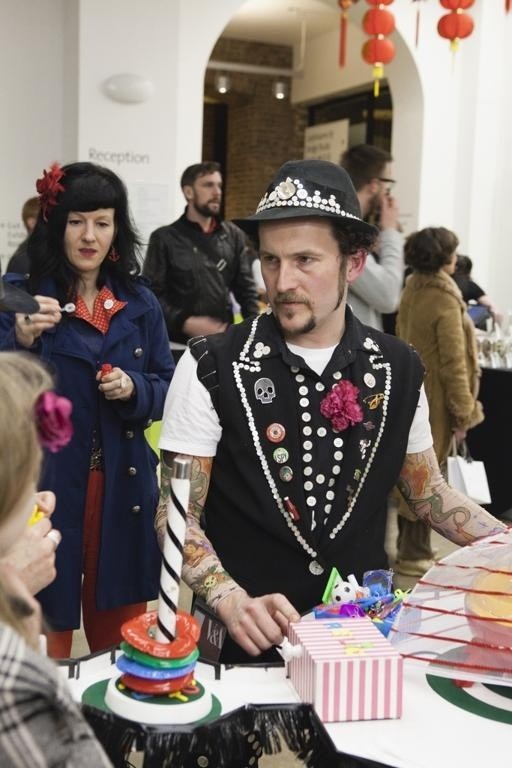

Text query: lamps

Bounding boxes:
[215,70,229,95]
[274,77,285,100]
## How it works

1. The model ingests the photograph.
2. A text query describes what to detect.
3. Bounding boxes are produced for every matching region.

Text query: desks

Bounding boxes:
[67,589,512,767]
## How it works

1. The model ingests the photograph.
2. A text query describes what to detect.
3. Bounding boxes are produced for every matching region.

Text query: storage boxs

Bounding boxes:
[284,620,402,726]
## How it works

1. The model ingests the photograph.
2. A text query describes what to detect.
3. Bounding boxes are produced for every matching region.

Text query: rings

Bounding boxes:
[121,376,126,389]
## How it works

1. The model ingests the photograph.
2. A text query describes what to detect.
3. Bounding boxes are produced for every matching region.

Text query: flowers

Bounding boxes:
[34,393,77,454]
[321,381,365,434]
[35,162,66,223]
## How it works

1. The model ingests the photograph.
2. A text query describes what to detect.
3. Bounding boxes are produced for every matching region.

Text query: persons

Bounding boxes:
[6,197,42,290]
[154,162,506,662]
[143,164,257,368]
[0,162,175,661]
[340,145,495,577]
[0,349,116,767]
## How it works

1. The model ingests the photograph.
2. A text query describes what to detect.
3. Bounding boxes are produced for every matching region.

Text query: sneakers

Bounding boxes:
[396,547,438,577]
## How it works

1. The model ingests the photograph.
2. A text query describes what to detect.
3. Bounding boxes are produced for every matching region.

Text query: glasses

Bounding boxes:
[369,177,396,190]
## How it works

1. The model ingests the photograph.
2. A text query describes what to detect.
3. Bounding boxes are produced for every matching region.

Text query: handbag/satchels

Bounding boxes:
[440,435,492,505]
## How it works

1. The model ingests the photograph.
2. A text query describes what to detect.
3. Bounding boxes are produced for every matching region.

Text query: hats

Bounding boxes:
[231,160,379,237]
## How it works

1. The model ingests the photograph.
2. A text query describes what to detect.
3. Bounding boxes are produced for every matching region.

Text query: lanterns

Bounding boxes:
[441,0,475,12]
[362,7,396,35]
[437,11,474,49]
[362,34,394,79]
[364,0,394,7]
